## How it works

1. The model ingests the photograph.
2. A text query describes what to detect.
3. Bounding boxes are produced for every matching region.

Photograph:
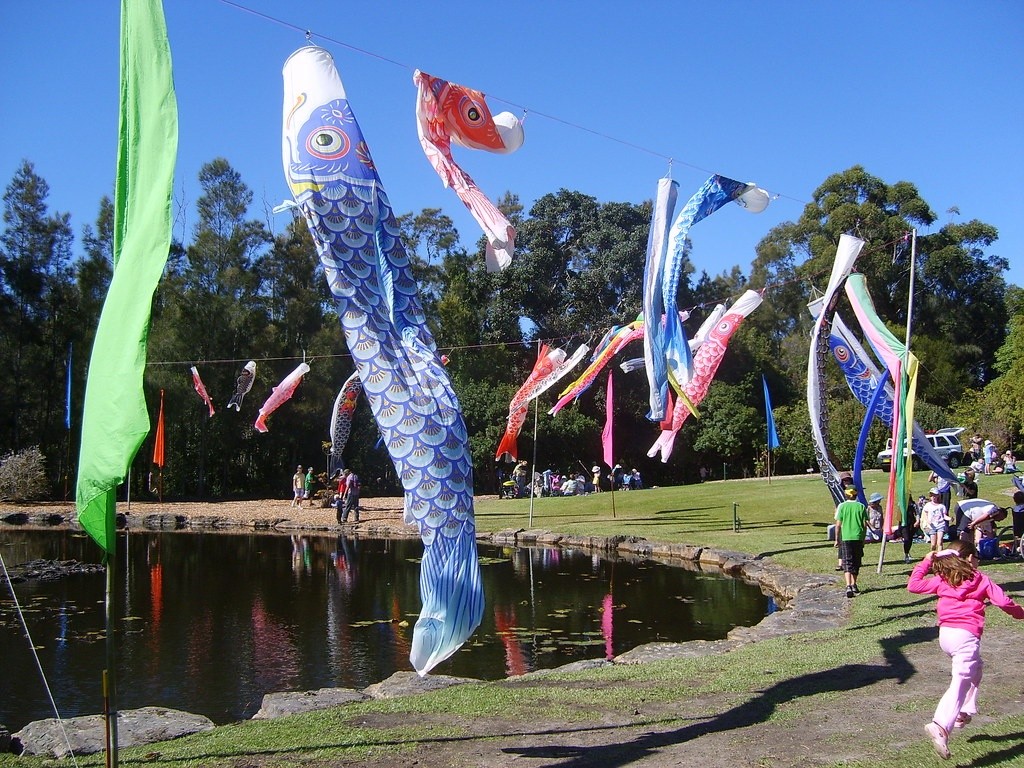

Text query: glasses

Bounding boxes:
[929,493,938,497]
[968,554,981,563]
[843,479,850,482]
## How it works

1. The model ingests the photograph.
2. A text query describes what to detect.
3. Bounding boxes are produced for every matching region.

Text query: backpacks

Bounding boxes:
[351,473,359,488]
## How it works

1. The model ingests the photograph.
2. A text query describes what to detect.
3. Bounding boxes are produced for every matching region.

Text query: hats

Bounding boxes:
[845,484,858,496]
[839,472,851,479]
[592,466,600,472]
[985,440,992,446]
[965,471,974,479]
[869,493,883,503]
[522,461,527,466]
[616,464,621,468]
[928,487,939,494]
[1006,450,1011,455]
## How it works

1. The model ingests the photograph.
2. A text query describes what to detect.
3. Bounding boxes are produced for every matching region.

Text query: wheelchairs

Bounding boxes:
[995,506,1024,561]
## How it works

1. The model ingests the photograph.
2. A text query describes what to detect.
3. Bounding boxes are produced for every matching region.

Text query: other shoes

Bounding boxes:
[905,553,913,564]
[836,565,845,570]
[852,583,859,592]
[846,586,852,598]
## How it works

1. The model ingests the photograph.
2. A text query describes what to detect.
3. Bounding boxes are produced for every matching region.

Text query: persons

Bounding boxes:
[700,465,709,483]
[291,465,363,524]
[900,469,978,563]
[507,461,586,497]
[828,473,884,596]
[963,434,1016,475]
[906,540,1023,761]
[955,490,1024,559]
[617,468,643,490]
[593,471,601,492]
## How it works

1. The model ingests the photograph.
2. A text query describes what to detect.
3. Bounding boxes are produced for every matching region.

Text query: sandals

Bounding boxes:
[925,722,951,760]
[954,712,971,728]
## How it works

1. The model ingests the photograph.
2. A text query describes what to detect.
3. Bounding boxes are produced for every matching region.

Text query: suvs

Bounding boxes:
[877,427,966,472]
